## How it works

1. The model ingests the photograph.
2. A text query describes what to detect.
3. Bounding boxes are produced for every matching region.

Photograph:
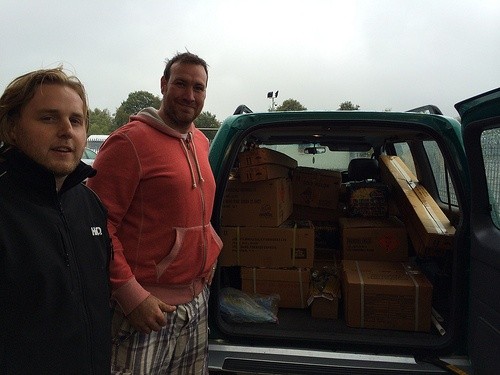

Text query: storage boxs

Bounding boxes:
[219,147,457,331]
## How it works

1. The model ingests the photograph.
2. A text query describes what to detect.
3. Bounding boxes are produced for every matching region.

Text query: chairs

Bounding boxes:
[345,158,391,217]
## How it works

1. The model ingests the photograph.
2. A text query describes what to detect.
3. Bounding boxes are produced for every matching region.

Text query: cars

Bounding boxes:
[80,147,98,184]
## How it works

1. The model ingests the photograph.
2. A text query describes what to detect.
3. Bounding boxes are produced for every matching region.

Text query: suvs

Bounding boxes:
[208,86,500,375]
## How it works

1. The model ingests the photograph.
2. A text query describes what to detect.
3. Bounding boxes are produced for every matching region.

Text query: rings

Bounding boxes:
[212,267,215,269]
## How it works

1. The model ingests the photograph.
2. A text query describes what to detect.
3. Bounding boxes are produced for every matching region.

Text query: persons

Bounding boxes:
[85,52,224,375]
[0,68,115,375]
